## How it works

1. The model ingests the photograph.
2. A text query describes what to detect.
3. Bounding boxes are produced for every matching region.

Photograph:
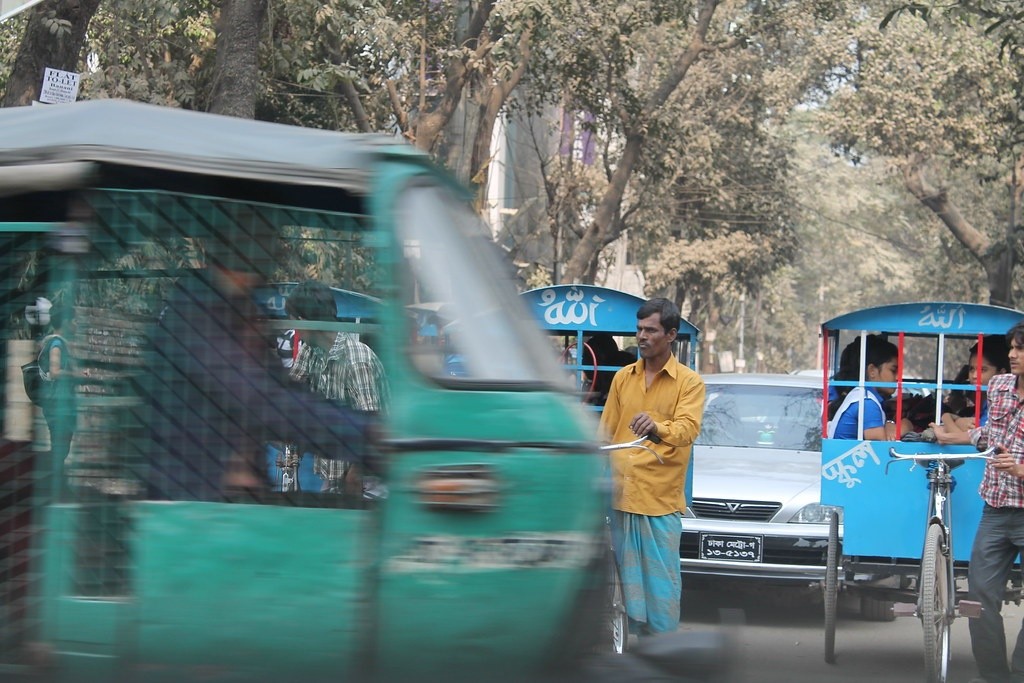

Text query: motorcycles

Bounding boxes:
[1,96,618,683]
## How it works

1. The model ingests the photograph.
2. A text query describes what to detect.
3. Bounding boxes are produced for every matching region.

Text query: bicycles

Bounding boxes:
[598,429,666,656]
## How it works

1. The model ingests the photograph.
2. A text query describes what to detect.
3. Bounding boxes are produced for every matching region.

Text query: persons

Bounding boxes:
[39,304,88,462]
[822,335,1011,442]
[268,281,387,497]
[595,299,705,630]
[931,322,1024,683]
[147,216,380,490]
[581,336,638,406]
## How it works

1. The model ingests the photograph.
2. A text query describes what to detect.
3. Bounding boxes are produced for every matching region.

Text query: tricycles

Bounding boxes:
[248,280,399,509]
[811,300,1023,683]
[436,282,703,520]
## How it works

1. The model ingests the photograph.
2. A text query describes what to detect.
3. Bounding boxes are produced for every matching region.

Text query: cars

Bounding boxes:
[676,371,900,625]
